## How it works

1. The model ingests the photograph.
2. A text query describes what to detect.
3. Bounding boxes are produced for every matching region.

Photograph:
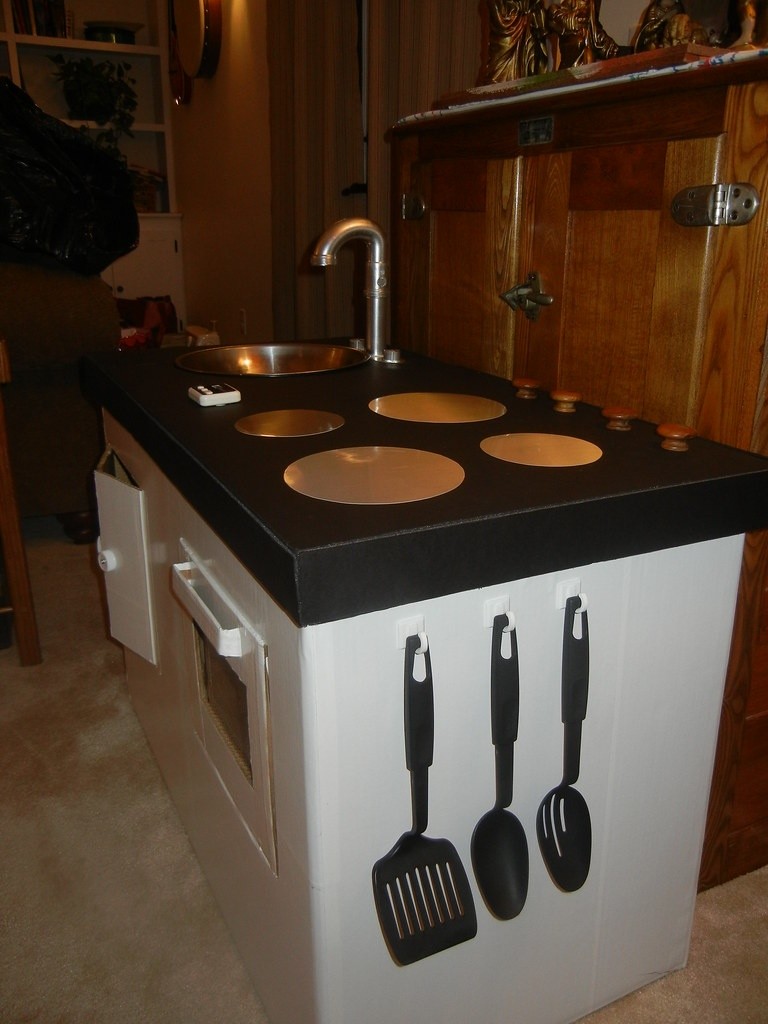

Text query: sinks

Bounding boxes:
[172,341,373,379]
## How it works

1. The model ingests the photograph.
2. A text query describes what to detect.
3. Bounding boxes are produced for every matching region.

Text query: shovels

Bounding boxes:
[369,631,477,966]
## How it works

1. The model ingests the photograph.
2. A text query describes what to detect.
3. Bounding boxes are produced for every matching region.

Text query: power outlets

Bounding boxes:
[238,310,247,336]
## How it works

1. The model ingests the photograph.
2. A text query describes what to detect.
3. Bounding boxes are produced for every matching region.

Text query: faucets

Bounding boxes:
[307,213,407,366]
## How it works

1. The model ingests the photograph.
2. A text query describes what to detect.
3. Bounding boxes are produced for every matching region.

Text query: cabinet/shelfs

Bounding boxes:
[0,0,189,333]
[375,39,768,895]
